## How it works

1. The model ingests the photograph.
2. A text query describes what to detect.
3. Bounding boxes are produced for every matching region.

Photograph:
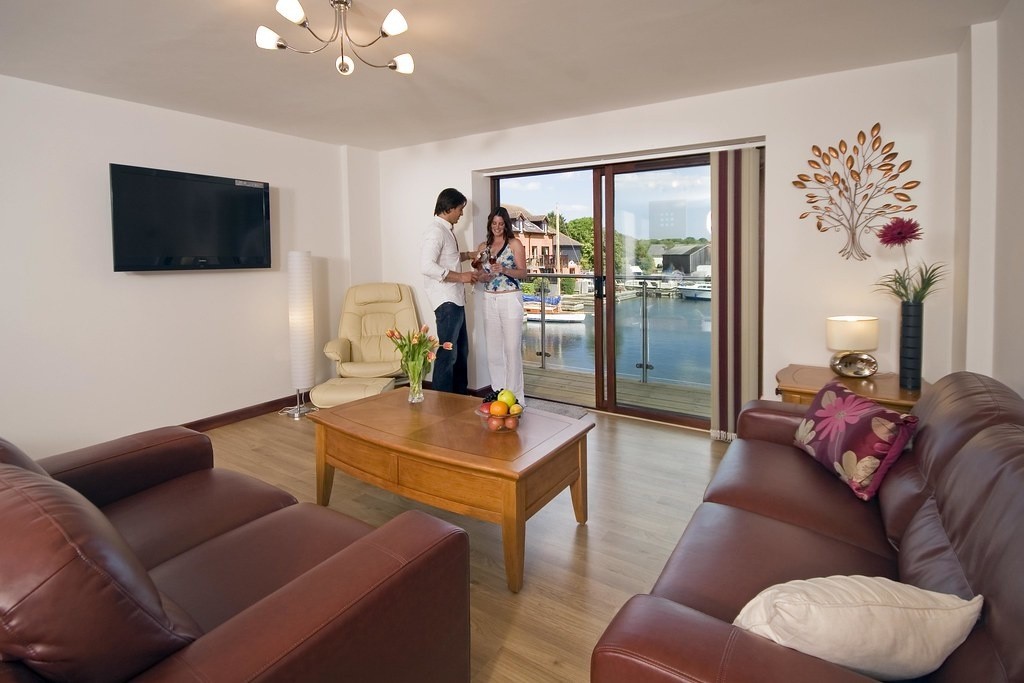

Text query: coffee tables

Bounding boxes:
[305,386,597,594]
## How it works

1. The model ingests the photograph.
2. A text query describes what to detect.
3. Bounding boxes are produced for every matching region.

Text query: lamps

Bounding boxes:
[255,0,414,75]
[285,249,314,418]
[824,316,879,378]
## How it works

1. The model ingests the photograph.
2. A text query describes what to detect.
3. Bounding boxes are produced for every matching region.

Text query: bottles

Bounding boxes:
[470,245,492,268]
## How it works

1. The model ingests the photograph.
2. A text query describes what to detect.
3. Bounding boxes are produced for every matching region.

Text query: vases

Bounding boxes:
[900,301,923,391]
[405,362,425,404]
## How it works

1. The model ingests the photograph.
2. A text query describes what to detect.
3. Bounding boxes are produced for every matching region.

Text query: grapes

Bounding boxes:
[483,389,519,405]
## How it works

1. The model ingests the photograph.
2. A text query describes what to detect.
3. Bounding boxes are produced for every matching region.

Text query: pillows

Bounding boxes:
[794,383,918,501]
[732,575,984,678]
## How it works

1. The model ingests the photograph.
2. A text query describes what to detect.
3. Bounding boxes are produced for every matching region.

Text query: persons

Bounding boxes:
[417,187,489,395]
[471,207,527,408]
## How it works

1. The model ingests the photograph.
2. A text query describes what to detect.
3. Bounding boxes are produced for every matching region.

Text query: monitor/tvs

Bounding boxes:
[109,163,271,273]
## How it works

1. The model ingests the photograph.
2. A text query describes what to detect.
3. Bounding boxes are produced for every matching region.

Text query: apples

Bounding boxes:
[505,414,519,429]
[488,417,504,431]
[498,390,516,408]
[509,404,523,416]
[480,402,492,420]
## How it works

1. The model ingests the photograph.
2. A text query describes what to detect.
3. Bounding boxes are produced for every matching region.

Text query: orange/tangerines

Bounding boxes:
[490,401,508,418]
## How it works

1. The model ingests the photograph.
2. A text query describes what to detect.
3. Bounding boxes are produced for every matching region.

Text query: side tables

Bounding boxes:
[777,363,933,414]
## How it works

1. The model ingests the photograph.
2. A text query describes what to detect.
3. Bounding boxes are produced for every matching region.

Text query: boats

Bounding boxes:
[677,265,712,300]
[522,294,586,324]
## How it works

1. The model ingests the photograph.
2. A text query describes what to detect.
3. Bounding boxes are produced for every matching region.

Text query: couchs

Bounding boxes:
[0,424,473,683]
[589,372,1024,683]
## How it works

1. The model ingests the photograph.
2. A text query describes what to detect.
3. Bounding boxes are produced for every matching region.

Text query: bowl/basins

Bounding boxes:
[474,409,523,434]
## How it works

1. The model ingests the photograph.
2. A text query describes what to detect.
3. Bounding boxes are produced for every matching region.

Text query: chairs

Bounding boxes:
[309,281,421,408]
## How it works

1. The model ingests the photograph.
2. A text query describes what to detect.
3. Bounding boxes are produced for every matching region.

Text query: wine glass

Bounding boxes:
[488,253,497,275]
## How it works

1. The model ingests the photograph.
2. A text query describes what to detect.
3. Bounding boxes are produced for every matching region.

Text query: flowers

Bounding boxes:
[872,218,950,303]
[385,325,454,362]
